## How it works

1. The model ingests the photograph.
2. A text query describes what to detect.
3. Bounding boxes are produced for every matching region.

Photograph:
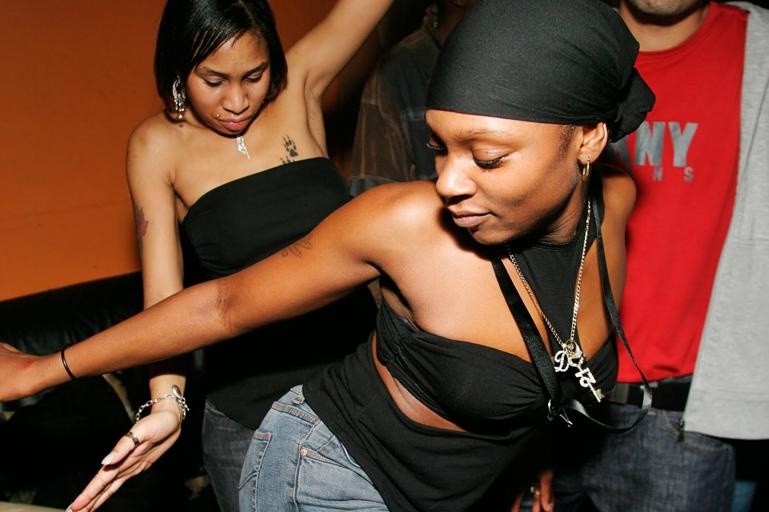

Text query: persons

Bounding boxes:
[345,0,484,310]
[0,0,660,512]
[63,0,396,511]
[556,2,768,511]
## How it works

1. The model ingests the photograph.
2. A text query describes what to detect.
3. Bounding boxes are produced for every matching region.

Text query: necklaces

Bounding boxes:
[232,132,253,160]
[503,191,608,406]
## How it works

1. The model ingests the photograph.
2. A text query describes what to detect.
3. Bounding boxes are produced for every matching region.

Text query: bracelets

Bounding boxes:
[59,345,75,383]
[133,382,191,424]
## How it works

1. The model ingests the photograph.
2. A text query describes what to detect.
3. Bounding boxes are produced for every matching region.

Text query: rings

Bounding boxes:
[527,485,542,500]
[122,430,140,447]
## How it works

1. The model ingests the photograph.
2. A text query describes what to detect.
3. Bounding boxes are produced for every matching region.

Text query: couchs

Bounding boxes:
[1,272,220,512]
